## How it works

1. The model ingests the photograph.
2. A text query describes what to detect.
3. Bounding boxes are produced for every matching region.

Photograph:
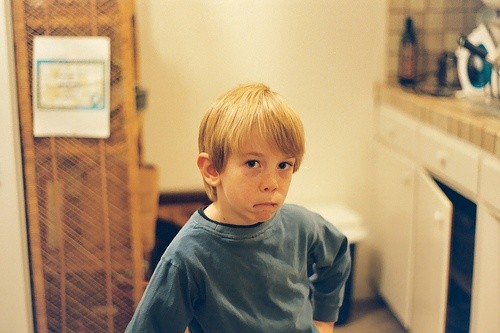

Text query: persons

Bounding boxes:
[125,85,352,333]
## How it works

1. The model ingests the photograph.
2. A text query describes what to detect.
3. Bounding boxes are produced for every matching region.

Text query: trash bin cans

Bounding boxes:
[308,204,365,326]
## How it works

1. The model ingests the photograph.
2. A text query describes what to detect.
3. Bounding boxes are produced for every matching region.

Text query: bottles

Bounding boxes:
[398,17,418,87]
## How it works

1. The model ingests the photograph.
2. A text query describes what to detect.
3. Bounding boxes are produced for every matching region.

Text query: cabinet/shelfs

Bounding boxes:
[11,0,161,333]
[374,103,500,333]
